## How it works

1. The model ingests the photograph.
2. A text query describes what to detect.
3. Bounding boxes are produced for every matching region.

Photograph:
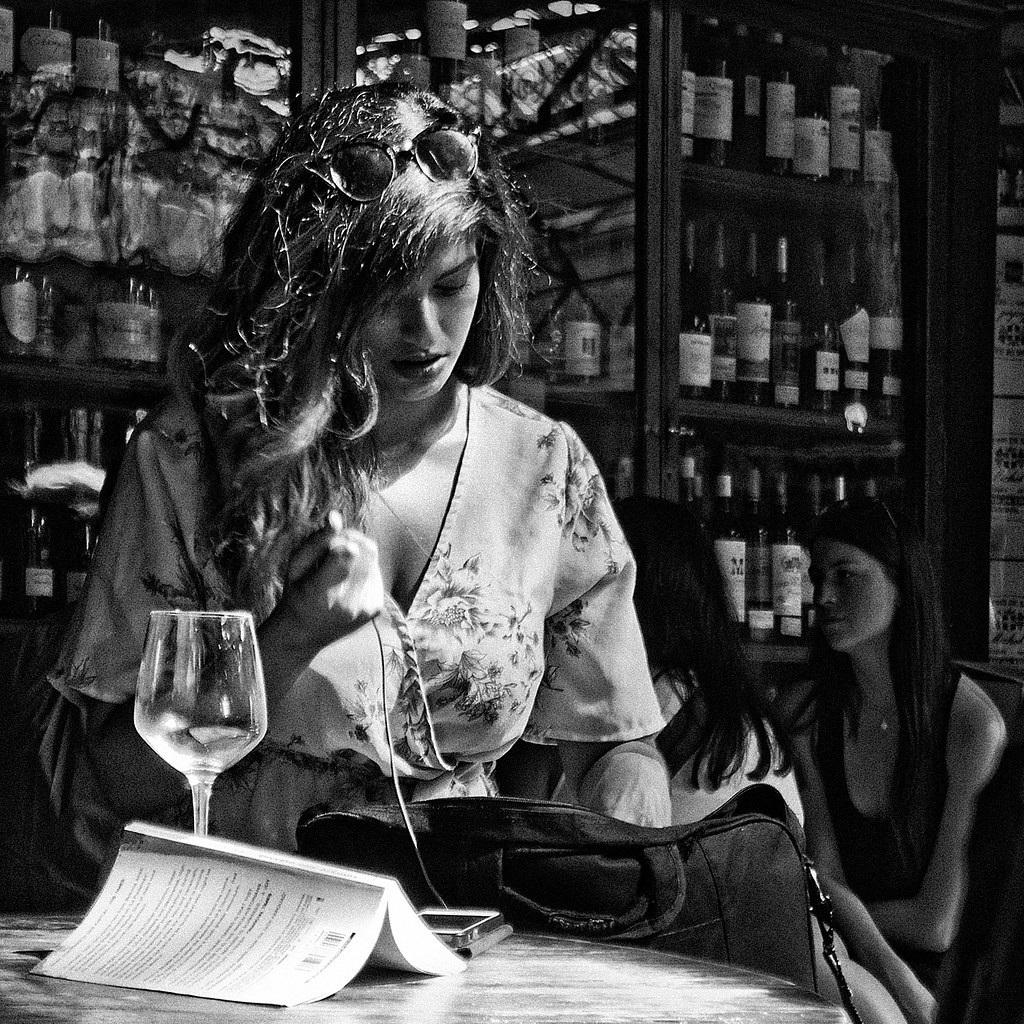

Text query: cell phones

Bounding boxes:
[418,907,503,947]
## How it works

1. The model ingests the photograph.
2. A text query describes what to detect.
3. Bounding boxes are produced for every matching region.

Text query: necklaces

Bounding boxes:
[862,705,896,730]
[371,481,434,561]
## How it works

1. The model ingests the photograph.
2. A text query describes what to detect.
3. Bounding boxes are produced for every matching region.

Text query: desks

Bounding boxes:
[0,893,873,1024]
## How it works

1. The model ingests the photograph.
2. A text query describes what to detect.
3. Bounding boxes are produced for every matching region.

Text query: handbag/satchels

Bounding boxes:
[296,786,865,1024]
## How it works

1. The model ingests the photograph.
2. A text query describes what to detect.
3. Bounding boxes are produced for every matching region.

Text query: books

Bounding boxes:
[29,821,470,1008]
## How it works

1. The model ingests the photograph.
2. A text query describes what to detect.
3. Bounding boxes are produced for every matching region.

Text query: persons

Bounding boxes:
[493,496,806,973]
[37,82,668,920]
[768,493,1007,1024]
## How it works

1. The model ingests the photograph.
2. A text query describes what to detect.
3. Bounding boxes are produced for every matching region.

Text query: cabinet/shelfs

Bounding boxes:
[0,0,339,753]
[317,0,944,736]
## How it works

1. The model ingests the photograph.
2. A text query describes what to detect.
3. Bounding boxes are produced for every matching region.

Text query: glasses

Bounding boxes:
[818,494,900,530]
[298,129,478,204]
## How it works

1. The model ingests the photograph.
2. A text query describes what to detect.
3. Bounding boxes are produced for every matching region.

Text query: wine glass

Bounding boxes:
[133,608,269,838]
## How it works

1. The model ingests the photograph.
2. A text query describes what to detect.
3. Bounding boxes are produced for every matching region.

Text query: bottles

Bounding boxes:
[0,1,908,652]
